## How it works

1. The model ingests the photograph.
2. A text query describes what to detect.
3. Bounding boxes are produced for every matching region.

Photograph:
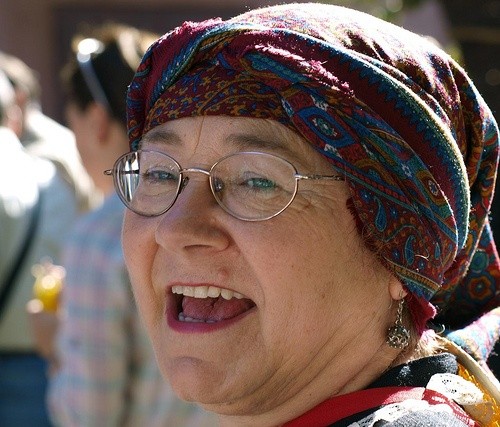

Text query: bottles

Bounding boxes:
[35,259,60,359]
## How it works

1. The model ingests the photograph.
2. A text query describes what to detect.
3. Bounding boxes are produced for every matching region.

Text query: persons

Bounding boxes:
[45,22,213,426]
[103,1,499,427]
[1,54,98,212]
[0,72,77,425]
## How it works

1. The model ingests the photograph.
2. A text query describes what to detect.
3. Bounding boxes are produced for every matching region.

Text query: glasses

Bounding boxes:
[104,150,343,222]
